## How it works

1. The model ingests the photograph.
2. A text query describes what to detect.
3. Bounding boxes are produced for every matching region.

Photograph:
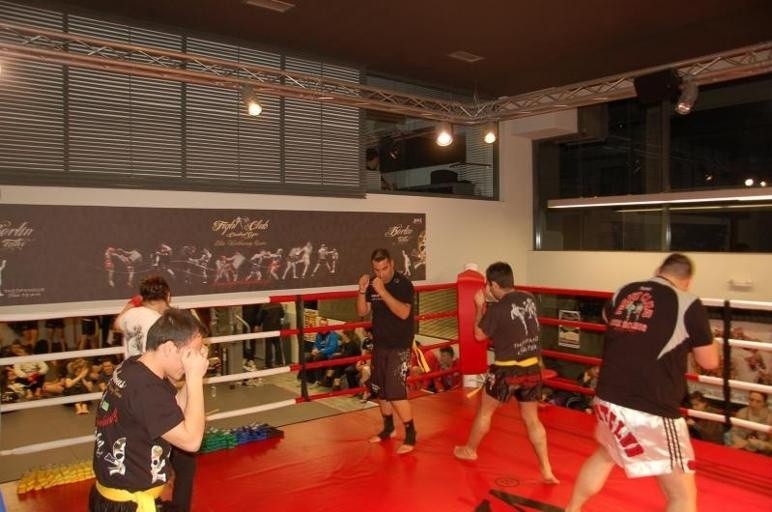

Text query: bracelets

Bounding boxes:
[358,289,369,294]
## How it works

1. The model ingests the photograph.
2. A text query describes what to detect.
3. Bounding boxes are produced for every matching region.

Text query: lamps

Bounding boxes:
[240,78,263,121]
[675,74,700,114]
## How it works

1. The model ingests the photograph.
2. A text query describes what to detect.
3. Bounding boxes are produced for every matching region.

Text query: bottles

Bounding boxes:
[210,385,216,398]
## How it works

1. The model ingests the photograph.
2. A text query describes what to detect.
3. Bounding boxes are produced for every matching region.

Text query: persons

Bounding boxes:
[686,391,725,444]
[112,275,196,512]
[400,249,412,277]
[366,148,393,191]
[412,230,426,278]
[452,261,561,485]
[295,318,376,403]
[242,304,285,369]
[731,390,771,452]
[356,248,419,455]
[104,241,340,290]
[1,315,123,415]
[541,359,552,399]
[582,366,599,389]
[564,251,721,512]
[406,341,463,393]
[88,307,211,512]
